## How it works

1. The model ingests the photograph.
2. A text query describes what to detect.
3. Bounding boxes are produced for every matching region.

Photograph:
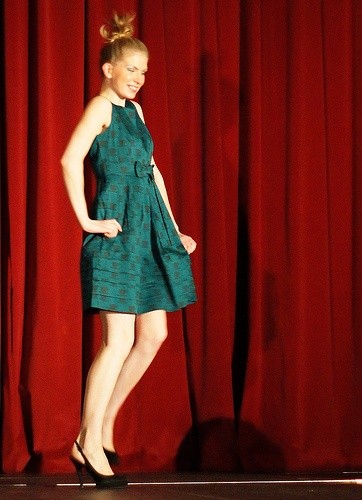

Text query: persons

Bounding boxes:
[60,6,197,493]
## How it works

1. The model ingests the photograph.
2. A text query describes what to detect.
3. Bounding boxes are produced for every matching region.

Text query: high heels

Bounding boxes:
[100,445,118,465]
[70,440,128,489]
